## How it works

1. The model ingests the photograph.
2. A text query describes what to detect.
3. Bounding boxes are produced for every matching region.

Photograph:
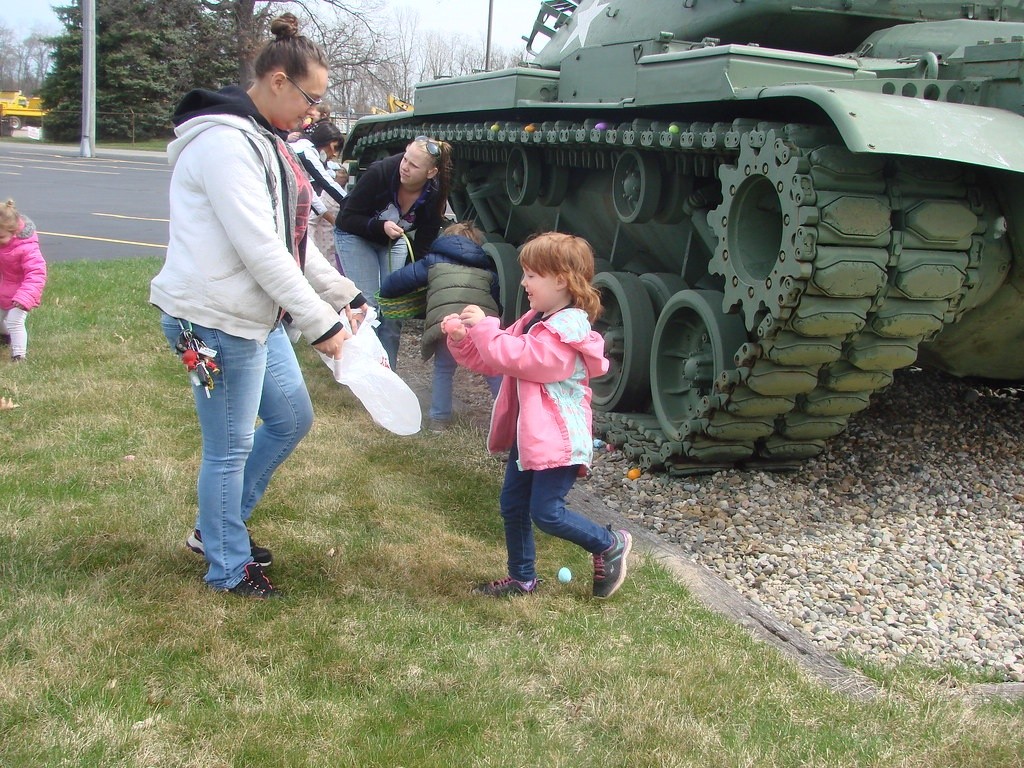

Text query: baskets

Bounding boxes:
[375,233,428,324]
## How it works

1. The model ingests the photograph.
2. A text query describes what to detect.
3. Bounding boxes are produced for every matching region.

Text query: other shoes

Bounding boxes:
[1,335,25,362]
[427,416,450,438]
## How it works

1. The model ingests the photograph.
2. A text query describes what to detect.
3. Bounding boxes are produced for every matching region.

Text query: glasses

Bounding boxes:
[414,134,442,158]
[284,74,322,107]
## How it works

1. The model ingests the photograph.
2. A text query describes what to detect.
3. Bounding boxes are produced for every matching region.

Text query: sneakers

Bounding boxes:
[227,563,282,598]
[471,577,537,600]
[186,524,272,567]
[592,528,632,602]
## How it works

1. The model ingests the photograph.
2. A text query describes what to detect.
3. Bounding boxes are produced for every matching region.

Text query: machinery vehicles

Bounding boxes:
[388,94,414,114]
[327,0,1024,477]
[371,105,390,115]
[0,91,49,130]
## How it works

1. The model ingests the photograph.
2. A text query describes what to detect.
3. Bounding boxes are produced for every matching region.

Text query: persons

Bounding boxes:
[0,197,46,360]
[307,163,348,269]
[442,232,633,600]
[379,218,511,429]
[274,100,330,143]
[149,11,377,603]
[333,134,453,376]
[286,121,348,227]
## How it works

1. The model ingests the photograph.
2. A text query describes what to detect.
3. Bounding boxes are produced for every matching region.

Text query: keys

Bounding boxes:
[175,329,220,399]
